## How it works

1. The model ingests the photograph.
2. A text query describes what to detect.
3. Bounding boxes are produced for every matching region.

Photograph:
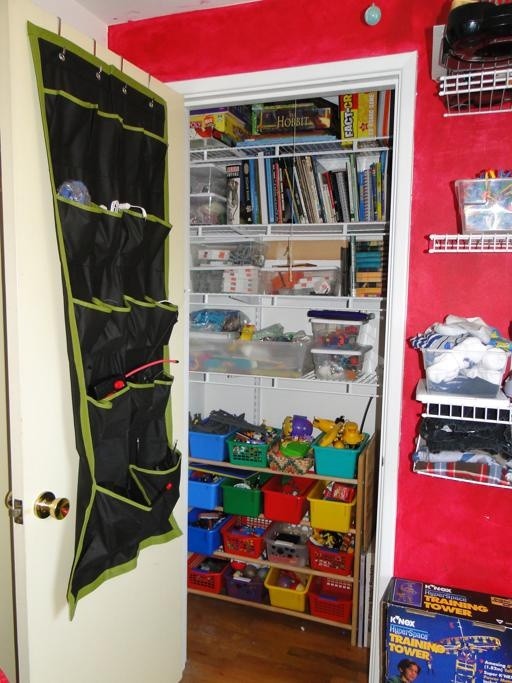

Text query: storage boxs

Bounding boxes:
[189,85,391,144]
[187,412,368,625]
[452,176,512,238]
[378,569,512,683]
[190,164,226,225]
[418,347,512,400]
[188,307,374,384]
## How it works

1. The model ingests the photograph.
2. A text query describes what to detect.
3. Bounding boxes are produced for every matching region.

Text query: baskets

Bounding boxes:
[305,575,353,624]
[225,428,282,467]
[188,552,231,594]
[267,442,314,475]
[220,516,354,576]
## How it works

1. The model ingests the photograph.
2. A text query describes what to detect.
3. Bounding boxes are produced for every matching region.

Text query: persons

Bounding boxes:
[385,658,422,682]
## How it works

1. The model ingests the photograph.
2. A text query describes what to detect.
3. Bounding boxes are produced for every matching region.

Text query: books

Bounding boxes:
[225,150,390,225]
[190,90,392,148]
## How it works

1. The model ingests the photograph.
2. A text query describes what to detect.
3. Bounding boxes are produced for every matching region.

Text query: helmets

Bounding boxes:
[439,3,512,110]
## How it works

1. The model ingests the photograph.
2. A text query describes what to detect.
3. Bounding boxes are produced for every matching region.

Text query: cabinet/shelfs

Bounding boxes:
[185,48,417,640]
[411,69,512,488]
